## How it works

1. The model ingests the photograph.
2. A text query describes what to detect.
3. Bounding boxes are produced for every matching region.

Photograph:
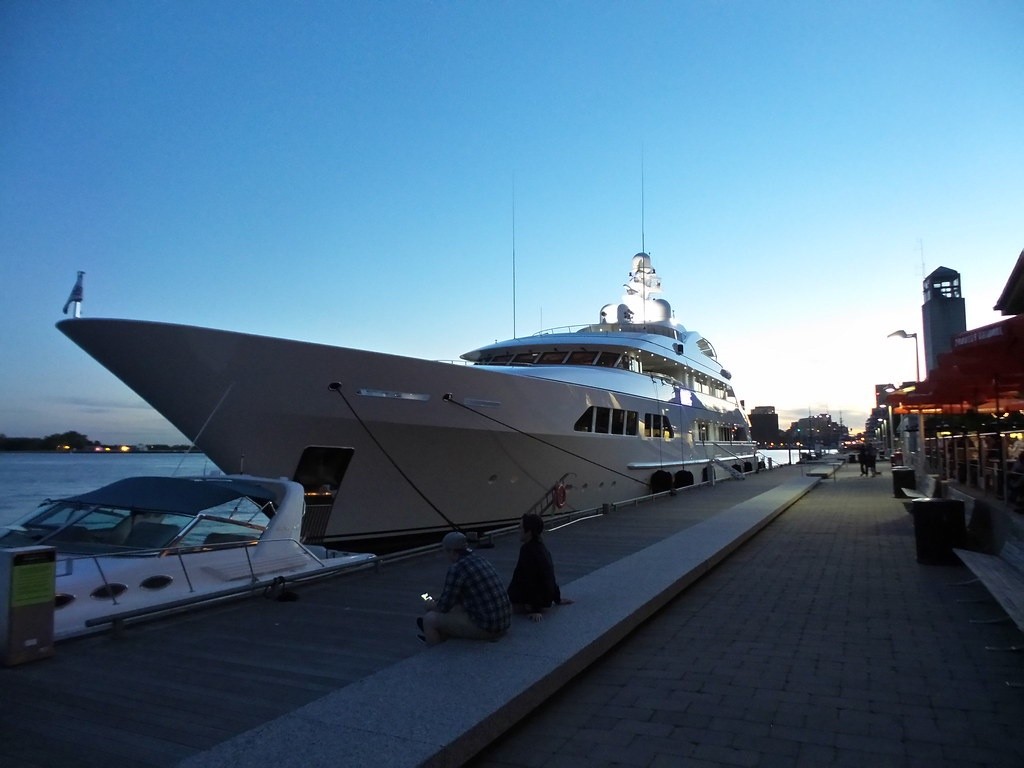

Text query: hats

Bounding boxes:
[442,531,473,555]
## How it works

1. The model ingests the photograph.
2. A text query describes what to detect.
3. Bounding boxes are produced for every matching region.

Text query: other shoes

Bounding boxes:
[416,634,432,648]
[416,617,424,633]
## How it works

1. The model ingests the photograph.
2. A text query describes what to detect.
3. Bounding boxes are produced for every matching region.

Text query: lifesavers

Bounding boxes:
[555,484,567,509]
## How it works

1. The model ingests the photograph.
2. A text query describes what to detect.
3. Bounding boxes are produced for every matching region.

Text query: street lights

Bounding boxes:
[887,329,926,474]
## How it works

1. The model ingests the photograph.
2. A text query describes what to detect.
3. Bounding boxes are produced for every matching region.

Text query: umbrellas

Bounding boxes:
[886,314,1024,497]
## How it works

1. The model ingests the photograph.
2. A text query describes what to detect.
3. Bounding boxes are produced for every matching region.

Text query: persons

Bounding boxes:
[858,443,878,478]
[422,532,512,649]
[415,513,575,644]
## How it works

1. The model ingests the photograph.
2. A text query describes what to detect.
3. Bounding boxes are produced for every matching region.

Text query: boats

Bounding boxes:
[0,384,378,645]
[56,133,757,555]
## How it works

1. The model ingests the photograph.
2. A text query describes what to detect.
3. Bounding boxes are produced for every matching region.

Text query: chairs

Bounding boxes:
[204,532,257,551]
[121,522,182,550]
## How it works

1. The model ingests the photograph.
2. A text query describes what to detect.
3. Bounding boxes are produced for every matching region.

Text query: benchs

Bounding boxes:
[953,542,1024,686]
[901,476,941,499]
